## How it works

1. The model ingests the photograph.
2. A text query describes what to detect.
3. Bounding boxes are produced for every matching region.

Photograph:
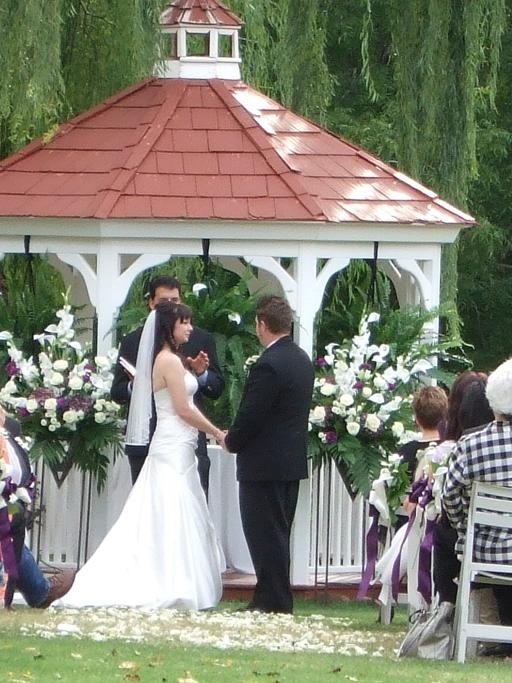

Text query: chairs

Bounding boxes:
[448,481,511,664]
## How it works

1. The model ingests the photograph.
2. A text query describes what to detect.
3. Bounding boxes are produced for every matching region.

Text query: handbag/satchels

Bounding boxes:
[398,601,453,660]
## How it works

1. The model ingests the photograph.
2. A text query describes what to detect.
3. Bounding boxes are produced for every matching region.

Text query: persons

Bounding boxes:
[216,292,315,616]
[429,356,512,659]
[145,300,227,610]
[110,274,226,510]
[374,383,449,546]
[0,406,78,609]
[403,370,494,522]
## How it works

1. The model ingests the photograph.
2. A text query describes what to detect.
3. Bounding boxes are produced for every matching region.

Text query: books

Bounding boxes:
[119,354,135,380]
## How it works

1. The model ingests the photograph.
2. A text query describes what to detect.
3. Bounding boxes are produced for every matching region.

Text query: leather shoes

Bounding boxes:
[42,568,76,608]
